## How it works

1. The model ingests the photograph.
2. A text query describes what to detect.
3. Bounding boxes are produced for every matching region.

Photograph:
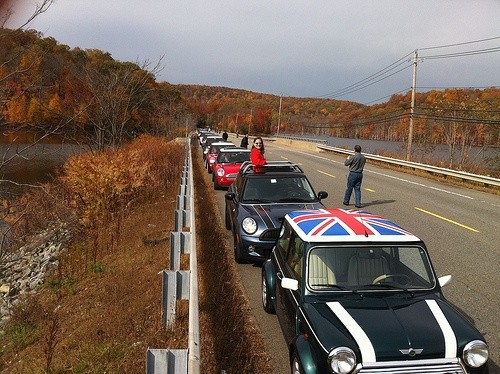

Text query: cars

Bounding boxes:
[195,127,254,190]
[262,206,491,374]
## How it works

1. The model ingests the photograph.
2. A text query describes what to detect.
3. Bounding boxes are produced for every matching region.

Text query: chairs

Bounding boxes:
[304,247,337,296]
[347,248,392,285]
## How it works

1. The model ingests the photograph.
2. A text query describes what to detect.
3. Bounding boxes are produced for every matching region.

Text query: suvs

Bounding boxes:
[224,160,328,264]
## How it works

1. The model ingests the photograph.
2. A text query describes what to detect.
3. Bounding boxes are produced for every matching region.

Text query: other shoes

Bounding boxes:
[343,202,349,205]
[355,204,361,208]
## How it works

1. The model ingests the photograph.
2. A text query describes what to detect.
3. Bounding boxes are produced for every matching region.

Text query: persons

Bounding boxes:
[240,134,249,149]
[250,138,267,198]
[210,125,212,131]
[343,145,366,208]
[236,131,240,138]
[222,131,228,142]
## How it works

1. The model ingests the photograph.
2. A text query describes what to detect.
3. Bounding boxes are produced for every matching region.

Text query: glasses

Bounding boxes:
[256,143,262,144]
